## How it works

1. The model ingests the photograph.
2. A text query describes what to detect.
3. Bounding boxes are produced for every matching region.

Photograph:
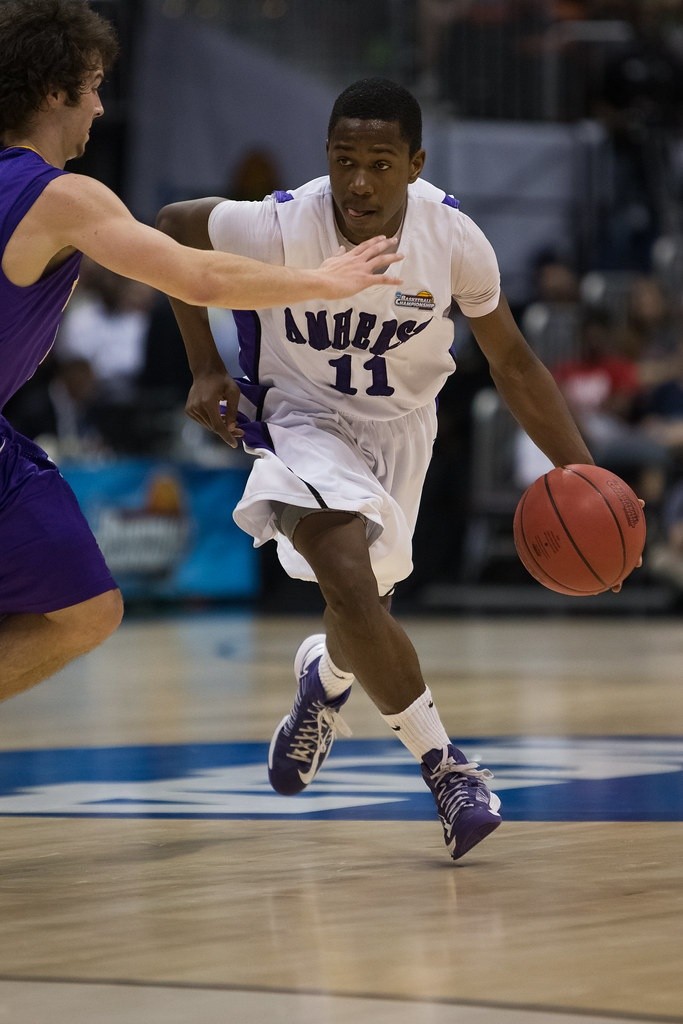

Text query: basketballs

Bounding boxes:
[512,464,647,596]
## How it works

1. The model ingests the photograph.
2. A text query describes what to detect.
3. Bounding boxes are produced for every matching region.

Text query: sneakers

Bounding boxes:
[420,743,502,861]
[267,632,355,796]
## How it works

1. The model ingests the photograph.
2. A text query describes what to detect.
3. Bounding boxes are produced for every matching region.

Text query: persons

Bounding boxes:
[152,73,650,863]
[3,138,682,612]
[0,1,407,711]
